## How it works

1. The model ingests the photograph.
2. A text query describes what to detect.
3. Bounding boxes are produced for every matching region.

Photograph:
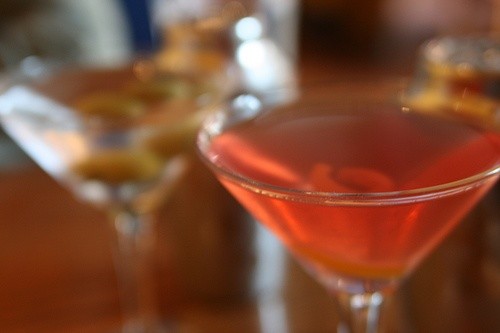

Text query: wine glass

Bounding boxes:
[194,72,500,333]
[1,57,237,333]
